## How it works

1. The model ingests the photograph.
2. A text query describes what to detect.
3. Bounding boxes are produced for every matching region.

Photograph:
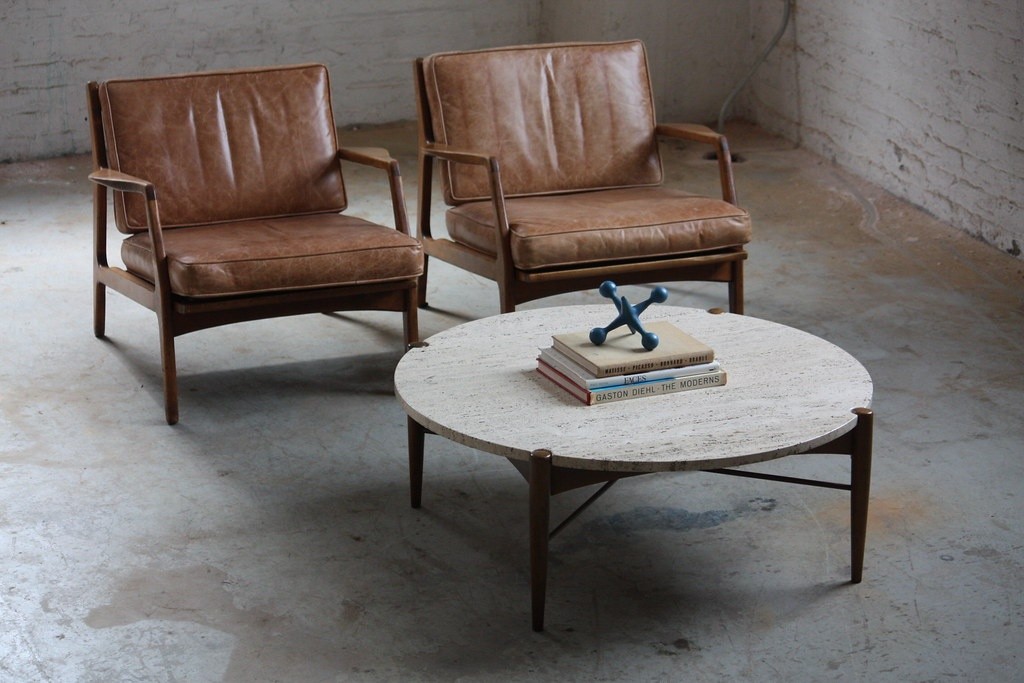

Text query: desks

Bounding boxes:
[393,281,874,633]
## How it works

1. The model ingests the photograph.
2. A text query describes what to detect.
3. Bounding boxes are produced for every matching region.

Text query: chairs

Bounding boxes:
[85,63,424,425]
[413,38,753,314]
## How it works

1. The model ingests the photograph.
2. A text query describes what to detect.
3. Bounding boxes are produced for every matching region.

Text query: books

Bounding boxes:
[536,318,727,405]
